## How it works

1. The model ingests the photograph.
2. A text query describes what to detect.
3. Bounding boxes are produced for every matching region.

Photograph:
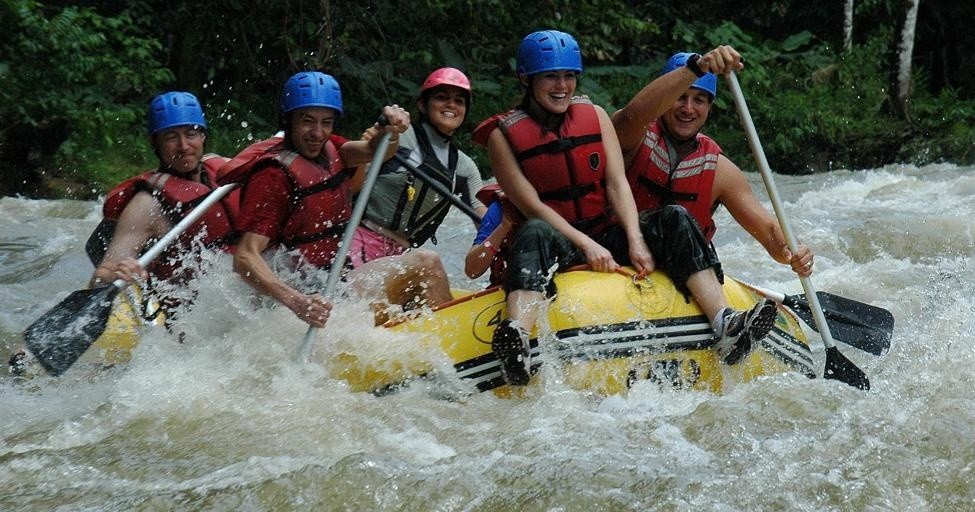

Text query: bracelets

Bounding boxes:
[484,239,496,255]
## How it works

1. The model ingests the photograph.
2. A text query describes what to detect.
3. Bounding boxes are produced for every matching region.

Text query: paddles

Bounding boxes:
[723,68,870,393]
[22,130,293,379]
[732,278,894,357]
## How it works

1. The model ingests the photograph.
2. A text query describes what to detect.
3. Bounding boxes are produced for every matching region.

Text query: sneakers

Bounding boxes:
[491,317,532,386]
[709,298,777,366]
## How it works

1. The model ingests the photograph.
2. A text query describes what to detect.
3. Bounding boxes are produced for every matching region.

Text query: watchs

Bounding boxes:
[687,54,707,78]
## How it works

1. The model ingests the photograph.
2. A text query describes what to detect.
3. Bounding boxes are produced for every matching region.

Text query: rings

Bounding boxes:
[806,261,811,269]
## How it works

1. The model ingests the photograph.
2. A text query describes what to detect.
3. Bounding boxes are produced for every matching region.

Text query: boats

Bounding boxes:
[57,266,817,416]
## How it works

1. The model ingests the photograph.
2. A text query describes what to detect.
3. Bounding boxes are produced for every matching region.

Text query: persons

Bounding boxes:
[89,45,511,324]
[471,29,776,386]
[612,45,815,276]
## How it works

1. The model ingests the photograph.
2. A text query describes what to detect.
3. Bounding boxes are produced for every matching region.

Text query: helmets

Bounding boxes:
[661,52,718,96]
[421,67,472,97]
[148,91,209,136]
[516,30,583,77]
[280,72,344,119]
